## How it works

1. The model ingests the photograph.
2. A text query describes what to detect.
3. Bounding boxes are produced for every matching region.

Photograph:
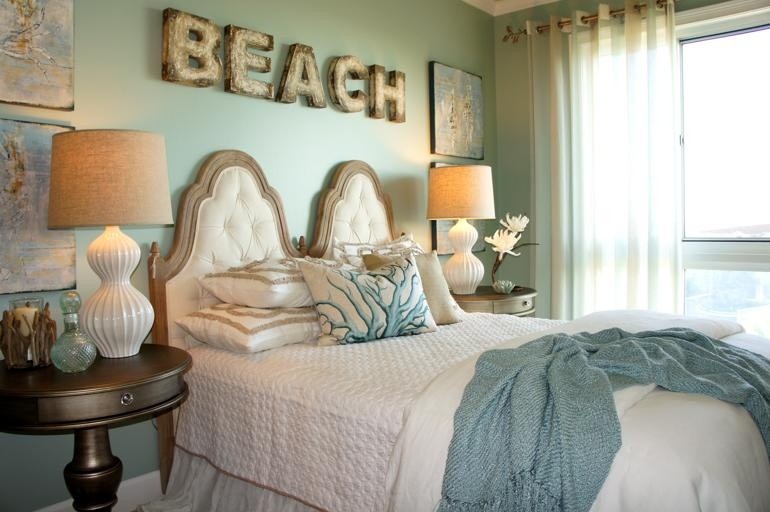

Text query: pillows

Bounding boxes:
[177,304,318,352]
[199,258,356,309]
[364,251,461,324]
[302,254,439,344]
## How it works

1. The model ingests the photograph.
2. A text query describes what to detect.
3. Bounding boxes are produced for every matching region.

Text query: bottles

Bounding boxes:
[51,292,97,373]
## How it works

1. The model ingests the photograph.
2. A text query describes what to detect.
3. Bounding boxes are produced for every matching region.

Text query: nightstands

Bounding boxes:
[448,287,539,318]
[1,344,193,511]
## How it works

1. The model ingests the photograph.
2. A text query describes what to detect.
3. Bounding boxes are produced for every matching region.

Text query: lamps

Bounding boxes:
[48,129,176,358]
[426,164,496,294]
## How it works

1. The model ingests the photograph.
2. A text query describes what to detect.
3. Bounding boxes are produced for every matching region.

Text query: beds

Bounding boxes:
[147,148,770,512]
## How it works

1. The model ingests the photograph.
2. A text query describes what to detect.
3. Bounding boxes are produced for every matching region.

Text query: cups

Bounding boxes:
[8,297,44,311]
